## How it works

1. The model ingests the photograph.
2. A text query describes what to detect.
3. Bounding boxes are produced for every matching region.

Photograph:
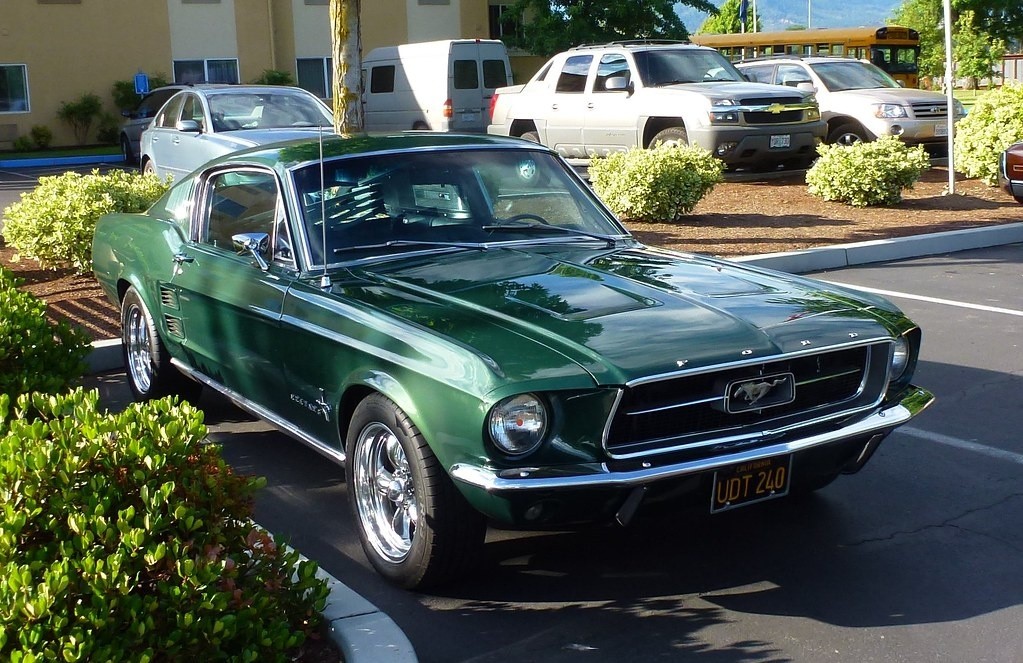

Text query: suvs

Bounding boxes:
[703,56,966,150]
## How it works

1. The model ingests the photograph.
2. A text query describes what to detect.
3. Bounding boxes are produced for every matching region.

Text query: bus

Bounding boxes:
[690,26,921,88]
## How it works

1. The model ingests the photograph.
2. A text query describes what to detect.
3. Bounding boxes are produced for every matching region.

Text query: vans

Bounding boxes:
[361,39,513,133]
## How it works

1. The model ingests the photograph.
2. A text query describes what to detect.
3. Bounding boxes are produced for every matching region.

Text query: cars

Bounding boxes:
[487,37,829,187]
[119,83,259,163]
[998,141,1023,205]
[91,130,936,589]
[139,85,335,185]
